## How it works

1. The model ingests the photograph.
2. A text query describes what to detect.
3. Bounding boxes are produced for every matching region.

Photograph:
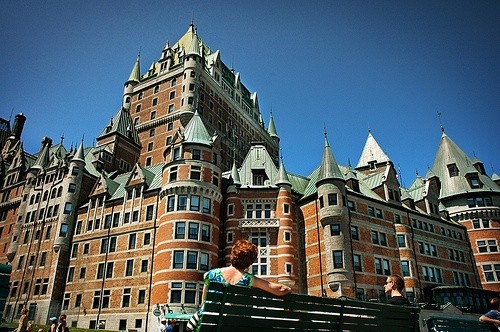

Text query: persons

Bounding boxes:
[374,274,412,327]
[159,319,176,332]
[479,298,500,331]
[50,316,65,332]
[196,239,292,332]
[14,308,29,332]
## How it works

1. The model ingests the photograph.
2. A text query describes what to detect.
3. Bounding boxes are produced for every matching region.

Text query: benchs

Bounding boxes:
[195,279,500,332]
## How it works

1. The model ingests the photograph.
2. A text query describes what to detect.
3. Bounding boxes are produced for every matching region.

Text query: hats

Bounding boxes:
[50,317,57,321]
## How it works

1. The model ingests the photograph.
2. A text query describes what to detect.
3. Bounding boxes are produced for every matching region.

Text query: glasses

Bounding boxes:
[385,281,398,285]
